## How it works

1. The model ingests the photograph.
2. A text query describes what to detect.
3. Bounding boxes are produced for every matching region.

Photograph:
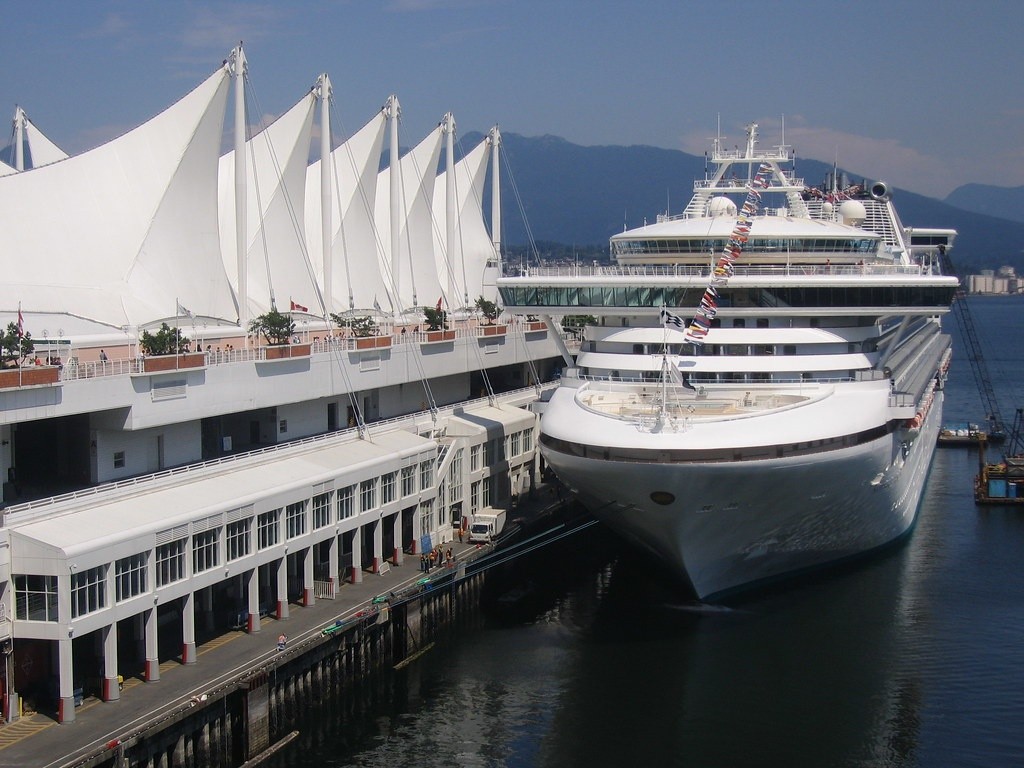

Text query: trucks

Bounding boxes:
[468,509,507,542]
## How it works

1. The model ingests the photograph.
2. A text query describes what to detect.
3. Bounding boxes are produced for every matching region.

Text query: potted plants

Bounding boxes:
[0,321,58,388]
[518,314,547,330]
[330,312,393,350]
[473,294,508,335]
[134,325,209,372]
[249,307,312,358]
[420,307,458,341]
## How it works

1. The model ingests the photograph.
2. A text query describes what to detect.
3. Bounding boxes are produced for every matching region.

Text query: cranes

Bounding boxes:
[938,242,1020,442]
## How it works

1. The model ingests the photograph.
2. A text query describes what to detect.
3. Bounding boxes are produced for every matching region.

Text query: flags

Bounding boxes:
[177,304,196,319]
[660,307,685,333]
[436,296,442,311]
[684,164,775,347]
[291,301,308,312]
[17,312,23,335]
[375,300,386,315]
[670,362,695,390]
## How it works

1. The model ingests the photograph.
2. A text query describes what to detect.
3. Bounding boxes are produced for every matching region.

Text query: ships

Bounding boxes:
[497,108,963,599]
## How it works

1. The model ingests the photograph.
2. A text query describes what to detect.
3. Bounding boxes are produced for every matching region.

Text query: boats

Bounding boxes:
[938,424,991,447]
[898,348,952,437]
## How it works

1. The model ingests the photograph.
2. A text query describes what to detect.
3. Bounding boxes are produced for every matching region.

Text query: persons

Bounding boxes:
[276,634,287,652]
[293,336,301,344]
[557,484,561,496]
[140,350,146,358]
[28,353,53,366]
[421,545,453,573]
[324,332,353,342]
[185,344,233,353]
[99,350,106,363]
[858,261,863,265]
[401,326,418,335]
[458,528,463,543]
[826,259,830,269]
